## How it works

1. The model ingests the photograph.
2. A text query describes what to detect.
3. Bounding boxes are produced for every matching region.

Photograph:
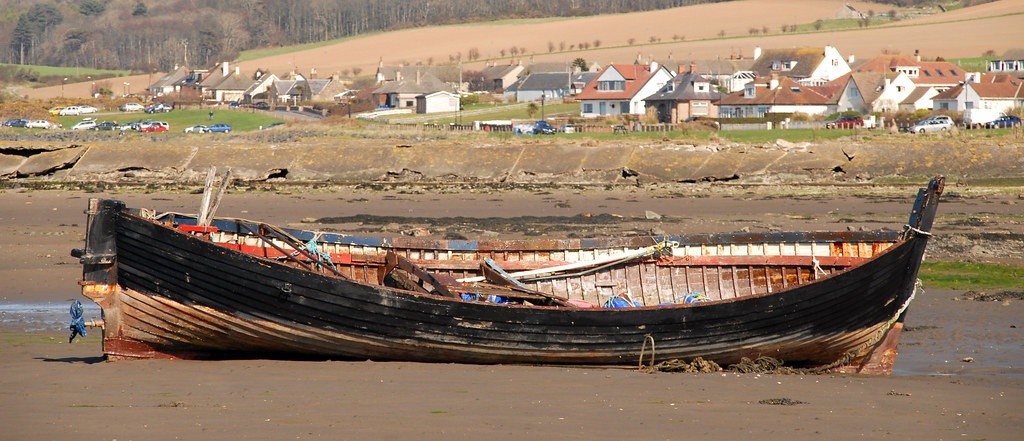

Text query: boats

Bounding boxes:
[65,169,949,382]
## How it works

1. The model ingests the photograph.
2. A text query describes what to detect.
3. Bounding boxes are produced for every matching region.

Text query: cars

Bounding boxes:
[143,103,172,114]
[185,125,206,134]
[559,124,575,134]
[48,105,99,116]
[254,102,269,109]
[532,120,557,135]
[73,122,96,130]
[229,102,240,110]
[118,102,144,112]
[203,124,233,134]
[118,120,170,133]
[907,115,955,134]
[983,115,1021,129]
[1,118,51,130]
[88,121,119,131]
[265,122,286,130]
[825,112,864,131]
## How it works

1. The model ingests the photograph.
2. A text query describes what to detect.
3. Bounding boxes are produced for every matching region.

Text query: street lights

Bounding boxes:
[61,78,68,98]
[87,75,95,98]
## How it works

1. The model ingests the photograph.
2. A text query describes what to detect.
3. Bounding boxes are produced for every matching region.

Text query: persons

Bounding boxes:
[209,110,214,120]
